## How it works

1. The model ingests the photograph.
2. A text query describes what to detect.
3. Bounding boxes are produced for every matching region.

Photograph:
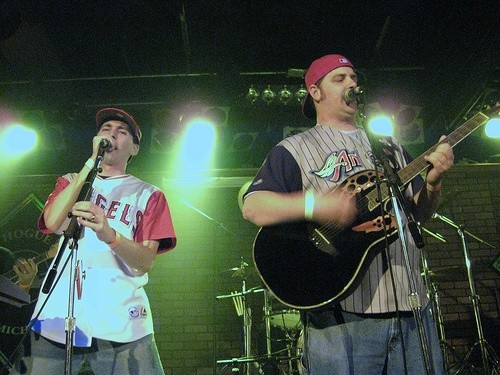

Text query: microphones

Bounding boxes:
[100,138,111,151]
[344,86,363,101]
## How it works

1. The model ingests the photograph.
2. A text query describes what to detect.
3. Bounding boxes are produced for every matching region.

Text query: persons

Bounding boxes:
[242,53,454,375]
[27,107,177,375]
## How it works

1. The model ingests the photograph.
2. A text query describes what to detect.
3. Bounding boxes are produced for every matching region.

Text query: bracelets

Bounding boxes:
[304,187,315,221]
[107,230,121,248]
[84,158,95,169]
[426,181,442,192]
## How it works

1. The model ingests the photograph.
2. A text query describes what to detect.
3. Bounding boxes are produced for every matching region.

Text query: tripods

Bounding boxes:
[214,286,301,375]
[416,213,500,375]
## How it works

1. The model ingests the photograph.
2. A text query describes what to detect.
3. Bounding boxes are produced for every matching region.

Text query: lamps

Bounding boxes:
[234,81,309,111]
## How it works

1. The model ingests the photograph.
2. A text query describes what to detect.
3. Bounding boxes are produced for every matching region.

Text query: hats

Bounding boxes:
[302,54,354,120]
[96,108,142,162]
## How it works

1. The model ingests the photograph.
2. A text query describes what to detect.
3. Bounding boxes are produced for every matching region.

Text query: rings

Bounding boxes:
[92,214,96,222]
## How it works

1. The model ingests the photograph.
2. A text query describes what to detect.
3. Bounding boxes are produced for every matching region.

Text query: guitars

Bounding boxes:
[254,102,500,310]
[2,242,59,280]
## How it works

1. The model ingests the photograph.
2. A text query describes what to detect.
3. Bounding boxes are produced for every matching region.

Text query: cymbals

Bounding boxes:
[222,267,257,283]
[421,265,459,275]
[269,309,299,330]
